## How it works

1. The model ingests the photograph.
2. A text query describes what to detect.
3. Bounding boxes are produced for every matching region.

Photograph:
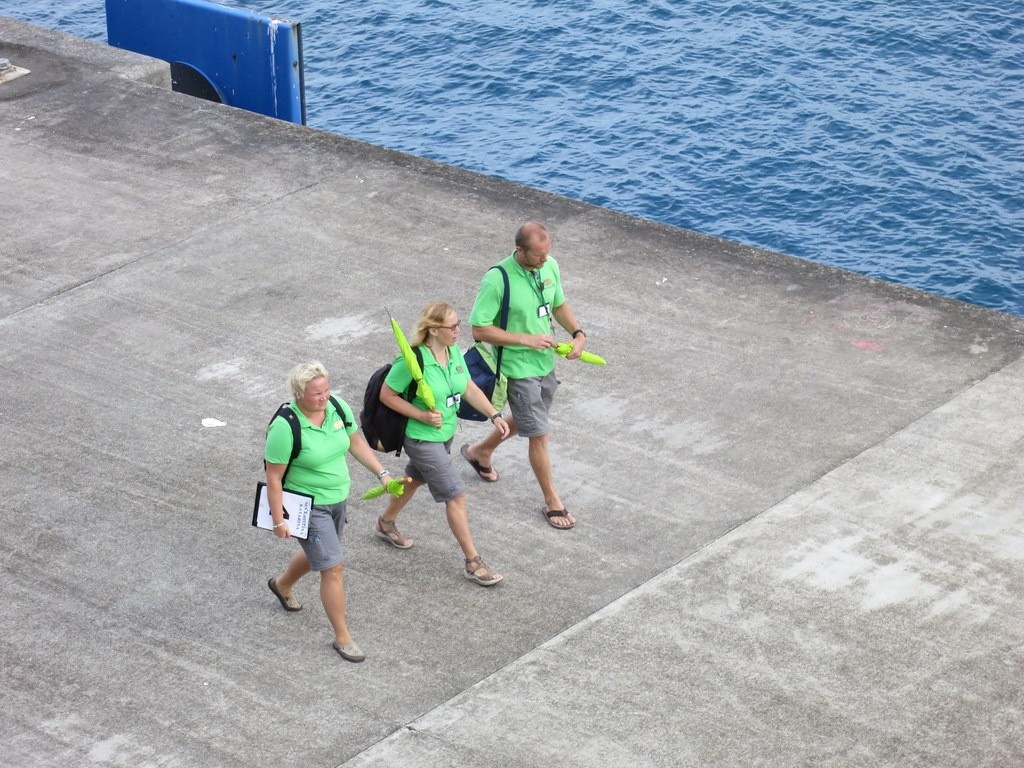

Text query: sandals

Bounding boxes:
[374,518,412,549]
[462,555,503,586]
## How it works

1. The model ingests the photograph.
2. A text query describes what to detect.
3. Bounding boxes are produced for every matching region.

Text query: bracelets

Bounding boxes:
[273,522,285,529]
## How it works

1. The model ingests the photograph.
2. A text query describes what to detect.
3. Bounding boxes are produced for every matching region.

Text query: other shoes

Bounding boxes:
[267,577,305,612]
[332,641,365,663]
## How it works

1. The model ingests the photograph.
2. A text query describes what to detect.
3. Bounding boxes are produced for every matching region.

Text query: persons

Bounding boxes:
[264,360,392,662]
[373,302,504,587]
[460,221,588,529]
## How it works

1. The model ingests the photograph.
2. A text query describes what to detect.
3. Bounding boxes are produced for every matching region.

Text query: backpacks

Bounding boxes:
[262,396,352,488]
[360,345,425,458]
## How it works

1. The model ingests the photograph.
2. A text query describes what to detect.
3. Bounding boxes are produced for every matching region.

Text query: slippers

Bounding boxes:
[542,504,576,529]
[461,444,500,483]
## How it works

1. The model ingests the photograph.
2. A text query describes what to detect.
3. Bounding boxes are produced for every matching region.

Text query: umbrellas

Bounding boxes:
[384,306,442,429]
[546,339,612,367]
[357,477,413,500]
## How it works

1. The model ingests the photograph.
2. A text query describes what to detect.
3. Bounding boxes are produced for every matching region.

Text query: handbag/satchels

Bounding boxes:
[456,342,509,422]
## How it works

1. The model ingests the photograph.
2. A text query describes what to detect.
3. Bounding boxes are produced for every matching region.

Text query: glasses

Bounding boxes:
[439,319,462,332]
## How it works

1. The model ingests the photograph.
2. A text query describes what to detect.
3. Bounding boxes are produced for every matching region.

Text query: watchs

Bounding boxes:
[490,412,503,424]
[572,330,586,338]
[378,469,390,480]
